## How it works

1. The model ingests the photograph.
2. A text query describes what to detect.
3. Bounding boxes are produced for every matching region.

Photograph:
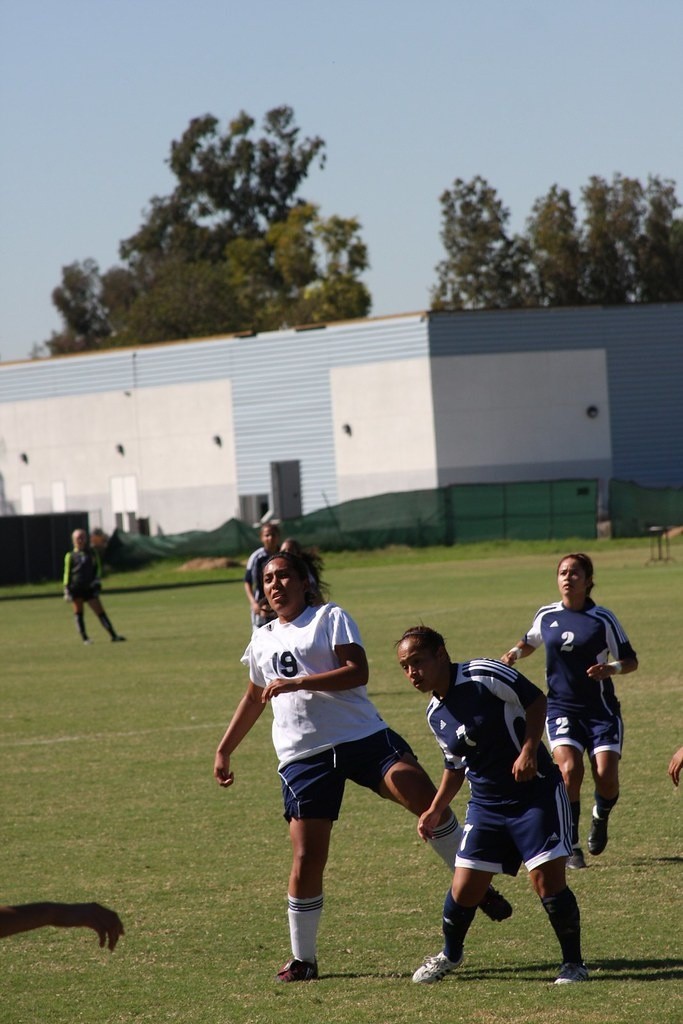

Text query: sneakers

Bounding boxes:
[412,950,464,984]
[553,962,588,984]
[566,848,586,869]
[587,805,608,855]
[274,956,318,982]
[479,889,511,920]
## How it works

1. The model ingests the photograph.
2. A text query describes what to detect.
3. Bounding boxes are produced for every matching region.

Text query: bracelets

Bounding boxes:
[509,647,521,660]
[609,662,621,675]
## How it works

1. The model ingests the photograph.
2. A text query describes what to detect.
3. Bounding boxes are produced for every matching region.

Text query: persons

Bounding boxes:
[242,519,326,631]
[62,529,128,646]
[1,902,125,952]
[214,547,639,986]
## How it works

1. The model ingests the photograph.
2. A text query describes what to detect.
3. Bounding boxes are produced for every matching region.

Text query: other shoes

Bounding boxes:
[111,636,125,642]
